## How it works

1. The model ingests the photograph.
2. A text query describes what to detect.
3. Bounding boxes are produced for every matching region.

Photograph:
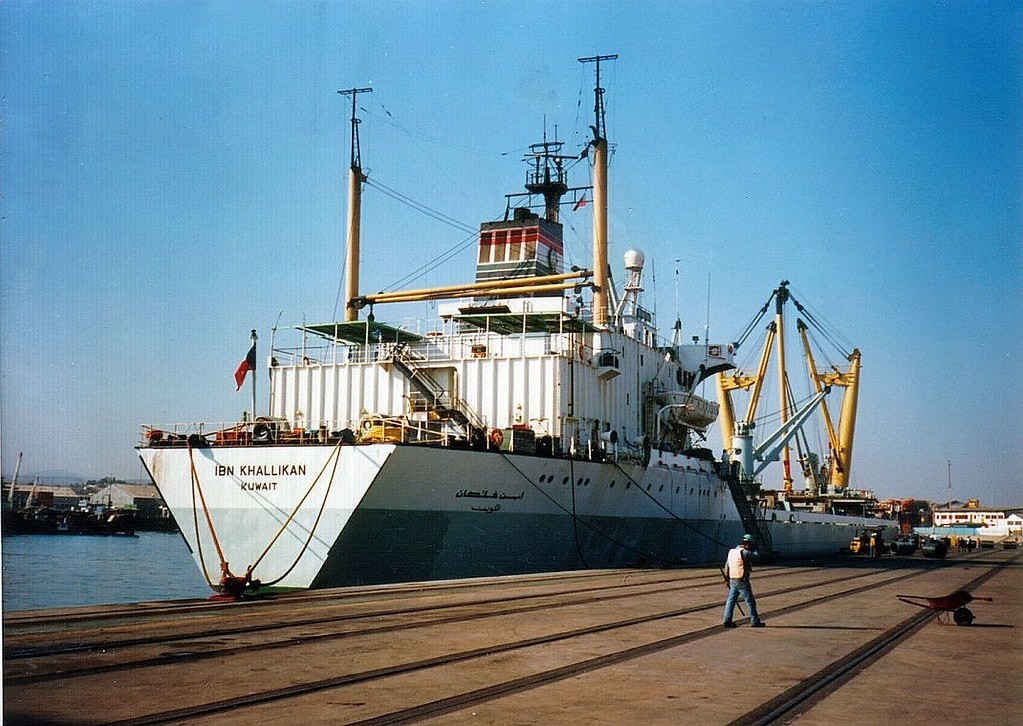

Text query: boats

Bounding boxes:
[132,46,904,610]
[656,389,721,433]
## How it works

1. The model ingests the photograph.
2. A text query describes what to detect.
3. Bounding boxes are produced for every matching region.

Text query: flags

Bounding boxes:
[234,343,256,390]
[573,195,586,211]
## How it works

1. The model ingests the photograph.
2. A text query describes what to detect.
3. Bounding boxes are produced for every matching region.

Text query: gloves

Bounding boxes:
[725,575,730,582]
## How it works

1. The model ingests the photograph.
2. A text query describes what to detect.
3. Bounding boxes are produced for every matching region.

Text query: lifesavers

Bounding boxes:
[491,430,503,446]
[710,346,719,356]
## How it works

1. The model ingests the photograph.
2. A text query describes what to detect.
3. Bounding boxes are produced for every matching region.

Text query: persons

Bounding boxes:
[721,534,765,629]
[966,536,972,554]
[721,449,729,463]
[869,533,876,557]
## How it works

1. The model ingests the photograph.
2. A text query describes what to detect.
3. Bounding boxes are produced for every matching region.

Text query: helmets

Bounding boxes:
[871,533,874,536]
[743,534,755,544]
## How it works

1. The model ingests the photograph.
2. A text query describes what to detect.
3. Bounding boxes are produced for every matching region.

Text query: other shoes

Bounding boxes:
[751,622,766,627]
[724,621,737,628]
